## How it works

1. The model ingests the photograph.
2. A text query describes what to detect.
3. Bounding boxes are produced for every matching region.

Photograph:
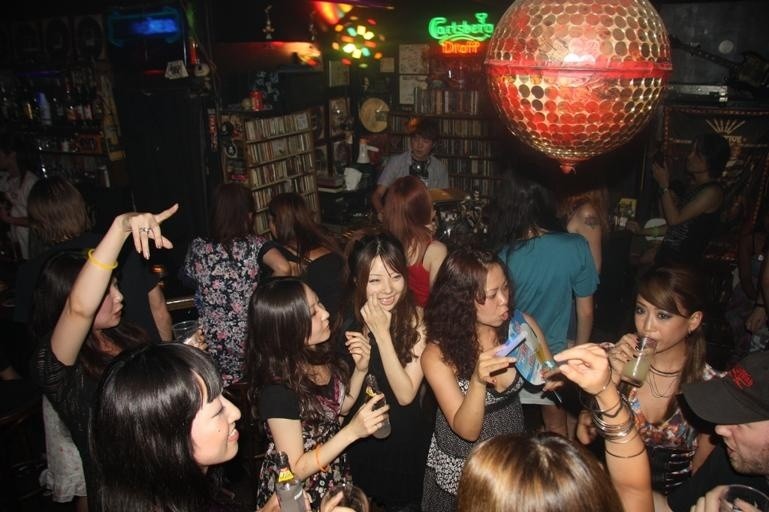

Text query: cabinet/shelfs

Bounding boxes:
[0,73,126,188]
[388,110,506,204]
[215,103,322,241]
[322,187,366,225]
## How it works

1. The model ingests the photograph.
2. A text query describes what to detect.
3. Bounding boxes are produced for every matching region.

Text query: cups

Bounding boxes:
[620,336,657,387]
[172,321,200,348]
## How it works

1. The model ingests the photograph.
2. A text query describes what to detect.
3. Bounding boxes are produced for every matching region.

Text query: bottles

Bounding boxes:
[721,484,768,512]
[274,452,303,512]
[1,80,111,189]
[365,373,392,440]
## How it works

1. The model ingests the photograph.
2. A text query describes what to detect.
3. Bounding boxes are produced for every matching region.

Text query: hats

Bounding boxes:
[680,348,769,426]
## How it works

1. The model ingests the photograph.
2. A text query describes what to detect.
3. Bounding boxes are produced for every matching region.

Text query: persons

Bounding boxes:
[0,116,769,511]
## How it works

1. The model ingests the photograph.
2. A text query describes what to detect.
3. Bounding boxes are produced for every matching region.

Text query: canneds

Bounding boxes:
[249,90,265,112]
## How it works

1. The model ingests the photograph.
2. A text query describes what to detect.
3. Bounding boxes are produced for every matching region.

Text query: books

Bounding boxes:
[419,91,494,196]
